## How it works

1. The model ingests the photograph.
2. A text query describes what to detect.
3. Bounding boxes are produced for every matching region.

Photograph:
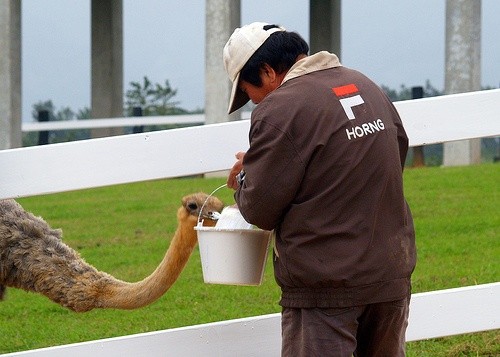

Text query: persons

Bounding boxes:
[222,22,417,356]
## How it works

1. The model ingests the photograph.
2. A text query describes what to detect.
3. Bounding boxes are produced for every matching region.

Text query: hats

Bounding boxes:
[222,21,285,116]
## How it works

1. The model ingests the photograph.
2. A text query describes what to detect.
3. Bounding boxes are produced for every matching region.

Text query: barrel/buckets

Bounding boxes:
[194,181,273,286]
